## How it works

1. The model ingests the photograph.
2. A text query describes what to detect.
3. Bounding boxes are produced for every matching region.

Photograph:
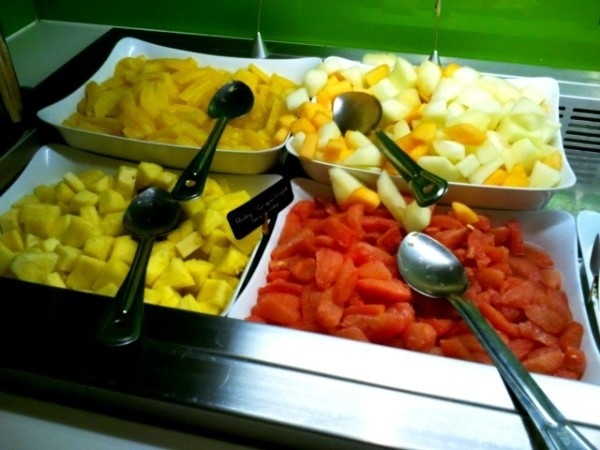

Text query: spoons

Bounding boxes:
[396,231,597,450]
[332,92,448,209]
[171,79,253,200]
[95,186,182,350]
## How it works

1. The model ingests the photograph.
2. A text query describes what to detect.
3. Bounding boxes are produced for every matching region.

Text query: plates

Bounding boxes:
[0,144,282,317]
[36,36,323,174]
[226,176,599,387]
[284,55,576,211]
